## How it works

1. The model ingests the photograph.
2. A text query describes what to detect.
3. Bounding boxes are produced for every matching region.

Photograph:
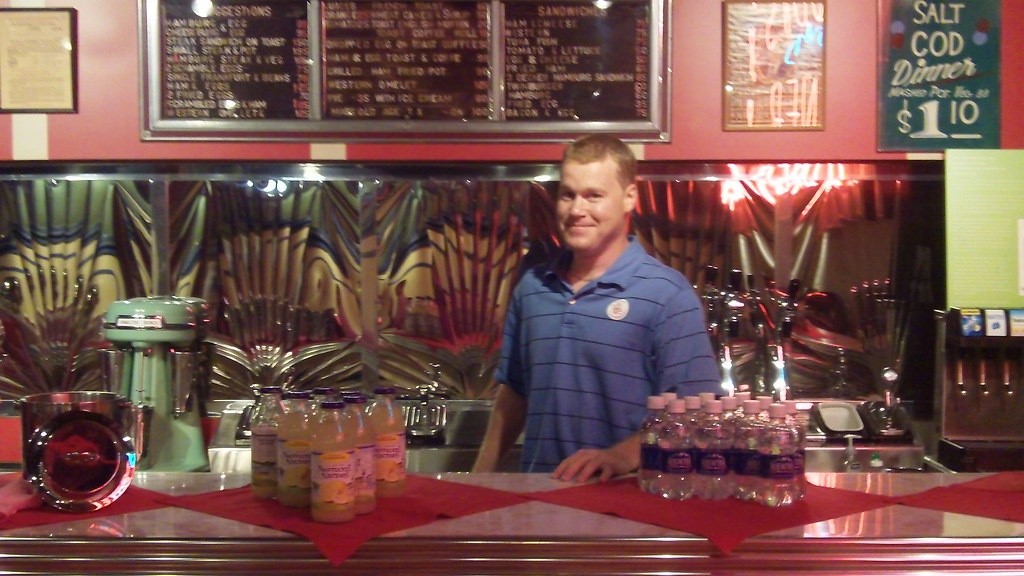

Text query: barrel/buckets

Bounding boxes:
[16,392,129,507]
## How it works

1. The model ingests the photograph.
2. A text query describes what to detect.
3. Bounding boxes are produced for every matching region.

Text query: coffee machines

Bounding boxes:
[100,294,211,471]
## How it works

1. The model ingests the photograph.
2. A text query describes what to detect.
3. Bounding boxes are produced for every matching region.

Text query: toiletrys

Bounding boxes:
[838,434,865,472]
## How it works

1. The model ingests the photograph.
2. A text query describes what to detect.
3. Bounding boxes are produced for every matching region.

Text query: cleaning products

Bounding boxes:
[868,452,885,472]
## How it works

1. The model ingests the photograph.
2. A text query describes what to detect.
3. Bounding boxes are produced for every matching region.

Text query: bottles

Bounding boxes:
[252,385,406,526]
[638,392,808,504]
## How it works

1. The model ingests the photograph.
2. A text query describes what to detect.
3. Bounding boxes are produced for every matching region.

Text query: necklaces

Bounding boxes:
[567,270,593,282]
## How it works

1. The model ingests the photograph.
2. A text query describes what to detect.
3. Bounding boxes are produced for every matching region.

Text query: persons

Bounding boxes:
[470,135,730,485]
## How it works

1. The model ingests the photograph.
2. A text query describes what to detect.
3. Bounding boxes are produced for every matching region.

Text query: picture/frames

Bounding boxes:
[0,7,78,114]
[721,0,828,131]
